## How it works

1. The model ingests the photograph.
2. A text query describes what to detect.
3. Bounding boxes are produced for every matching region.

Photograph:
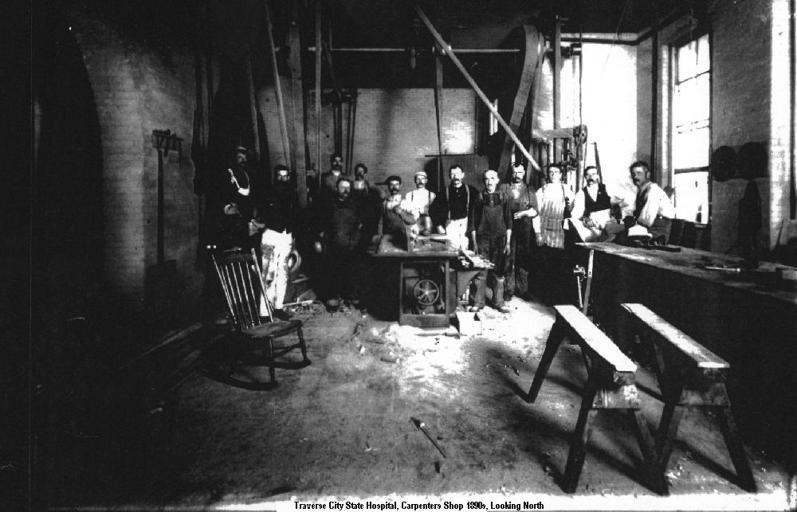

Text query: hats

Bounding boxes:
[415,172,427,177]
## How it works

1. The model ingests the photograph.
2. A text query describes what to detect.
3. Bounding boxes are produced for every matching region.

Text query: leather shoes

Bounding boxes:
[470,306,479,312]
[504,294,511,301]
[500,306,510,313]
[521,293,530,301]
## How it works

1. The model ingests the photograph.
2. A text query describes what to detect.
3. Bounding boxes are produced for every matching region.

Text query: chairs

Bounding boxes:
[207,245,311,390]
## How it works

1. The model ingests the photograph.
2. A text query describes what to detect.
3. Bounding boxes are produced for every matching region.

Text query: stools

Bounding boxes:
[619,303,758,492]
[527,304,669,495]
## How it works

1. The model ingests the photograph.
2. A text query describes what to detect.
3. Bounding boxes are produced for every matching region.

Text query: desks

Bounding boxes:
[574,242,797,315]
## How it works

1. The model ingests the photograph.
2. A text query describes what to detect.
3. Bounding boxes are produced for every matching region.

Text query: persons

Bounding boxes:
[215,145,673,324]
